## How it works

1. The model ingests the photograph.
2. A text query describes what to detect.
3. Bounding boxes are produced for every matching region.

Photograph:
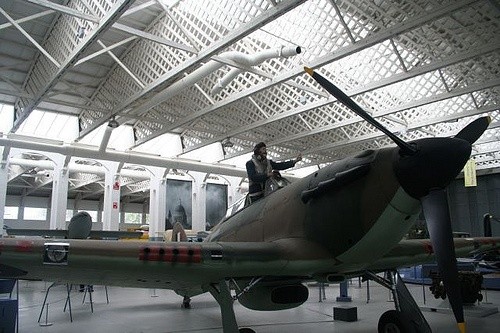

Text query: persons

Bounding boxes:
[245,142,302,204]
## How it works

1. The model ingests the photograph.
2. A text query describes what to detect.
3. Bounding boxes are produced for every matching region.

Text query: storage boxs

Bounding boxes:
[333,306,357,322]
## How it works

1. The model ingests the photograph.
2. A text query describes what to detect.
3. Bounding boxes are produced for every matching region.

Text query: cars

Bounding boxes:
[456,248,500,289]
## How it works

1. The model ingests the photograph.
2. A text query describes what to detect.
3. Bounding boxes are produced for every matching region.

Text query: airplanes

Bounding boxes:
[0,66,499,333]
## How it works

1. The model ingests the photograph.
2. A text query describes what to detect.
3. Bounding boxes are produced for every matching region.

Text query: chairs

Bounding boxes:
[0,211,500,323]
